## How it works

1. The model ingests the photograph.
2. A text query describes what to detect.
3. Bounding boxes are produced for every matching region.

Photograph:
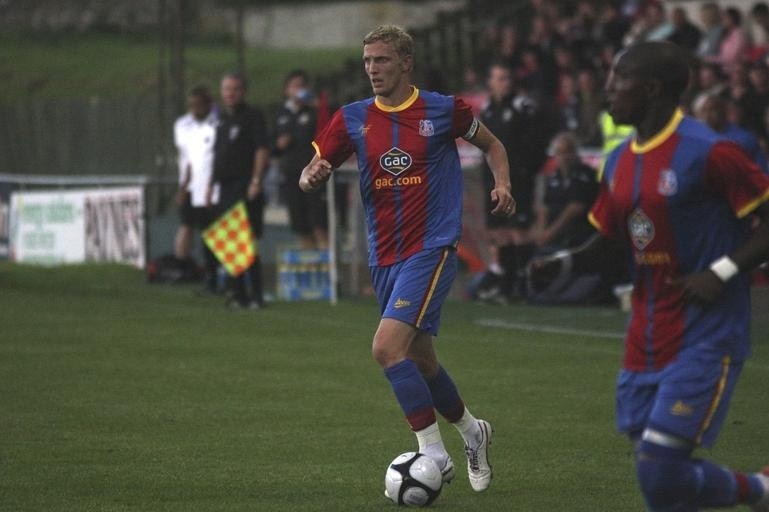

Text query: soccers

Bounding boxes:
[386,453,443,505]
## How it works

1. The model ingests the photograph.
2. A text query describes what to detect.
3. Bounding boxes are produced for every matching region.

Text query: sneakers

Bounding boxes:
[465,418,493,491]
[383,450,454,499]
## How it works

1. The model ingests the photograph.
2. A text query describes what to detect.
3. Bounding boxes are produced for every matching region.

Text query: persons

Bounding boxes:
[173,69,329,310]
[527,40,768,512]
[297,22,520,499]
[468,0,769,305]
